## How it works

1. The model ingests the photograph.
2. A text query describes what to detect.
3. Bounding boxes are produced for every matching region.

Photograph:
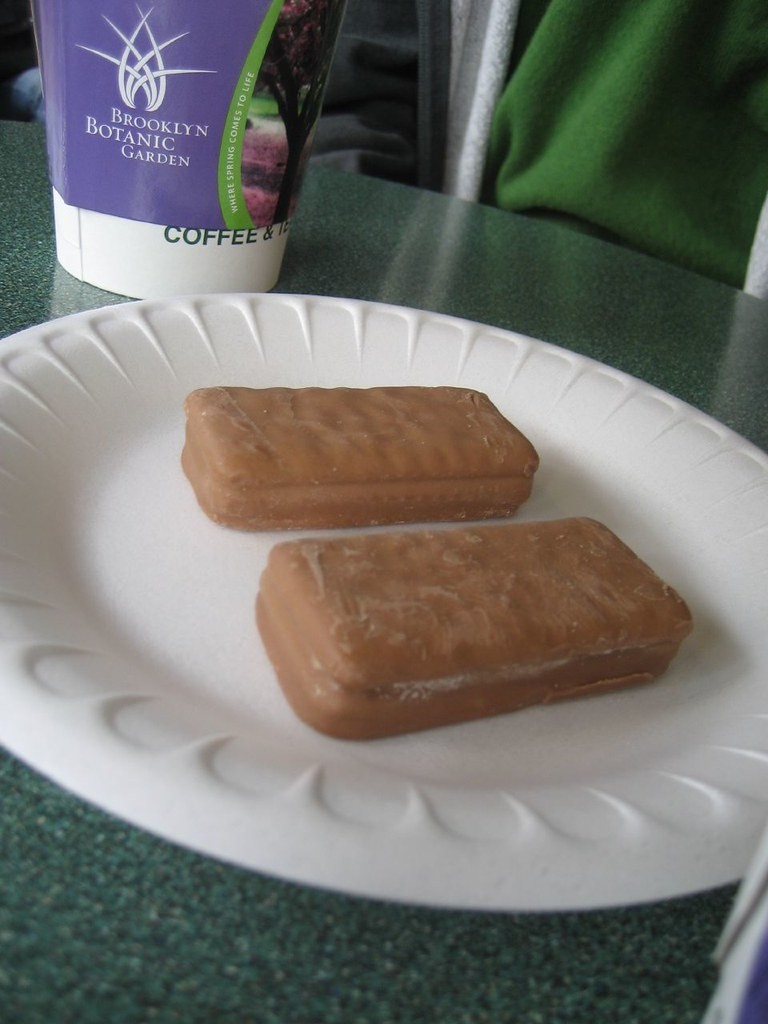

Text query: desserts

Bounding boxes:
[258,516,692,746]
[178,386,542,534]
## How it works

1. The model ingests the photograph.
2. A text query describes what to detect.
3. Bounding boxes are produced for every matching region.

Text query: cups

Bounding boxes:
[32,0,349,299]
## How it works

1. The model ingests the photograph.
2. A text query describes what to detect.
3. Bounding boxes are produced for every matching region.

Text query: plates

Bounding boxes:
[0,292,768,913]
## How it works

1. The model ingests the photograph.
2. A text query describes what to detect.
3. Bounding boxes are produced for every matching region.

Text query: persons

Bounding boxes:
[0,0,45,124]
[308,0,768,300]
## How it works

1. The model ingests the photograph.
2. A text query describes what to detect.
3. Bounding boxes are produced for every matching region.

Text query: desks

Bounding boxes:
[0,111,768,1024]
